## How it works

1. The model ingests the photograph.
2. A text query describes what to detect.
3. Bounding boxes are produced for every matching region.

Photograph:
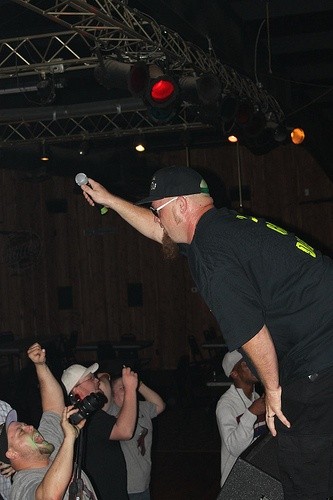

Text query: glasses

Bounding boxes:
[72,374,95,391]
[149,196,178,218]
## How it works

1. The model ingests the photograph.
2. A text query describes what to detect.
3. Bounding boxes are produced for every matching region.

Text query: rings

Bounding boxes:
[268,416,274,418]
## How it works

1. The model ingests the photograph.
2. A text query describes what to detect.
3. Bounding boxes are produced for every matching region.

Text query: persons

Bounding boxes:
[80,164,333,500]
[0,343,86,500]
[61,362,138,500]
[107,367,165,500]
[216,349,266,488]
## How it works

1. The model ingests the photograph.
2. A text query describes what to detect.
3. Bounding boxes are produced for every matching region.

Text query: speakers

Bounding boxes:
[217,431,286,500]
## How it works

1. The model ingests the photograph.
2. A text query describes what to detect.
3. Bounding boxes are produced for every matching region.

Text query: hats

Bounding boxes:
[221,350,243,378]
[62,363,100,396]
[133,165,207,205]
[0,409,17,464]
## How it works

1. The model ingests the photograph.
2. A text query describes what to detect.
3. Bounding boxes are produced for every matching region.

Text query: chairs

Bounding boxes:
[0,327,224,432]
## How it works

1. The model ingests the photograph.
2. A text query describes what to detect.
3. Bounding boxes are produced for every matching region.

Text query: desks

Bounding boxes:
[0,341,25,377]
[205,382,232,418]
[201,343,226,367]
[76,343,145,350]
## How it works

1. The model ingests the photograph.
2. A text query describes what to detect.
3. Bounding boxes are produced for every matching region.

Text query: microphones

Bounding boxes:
[75,172,108,216]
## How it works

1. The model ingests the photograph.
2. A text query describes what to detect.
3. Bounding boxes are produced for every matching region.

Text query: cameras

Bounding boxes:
[68,392,108,426]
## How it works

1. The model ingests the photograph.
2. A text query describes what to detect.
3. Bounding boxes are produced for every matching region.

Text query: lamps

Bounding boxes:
[92,46,305,156]
